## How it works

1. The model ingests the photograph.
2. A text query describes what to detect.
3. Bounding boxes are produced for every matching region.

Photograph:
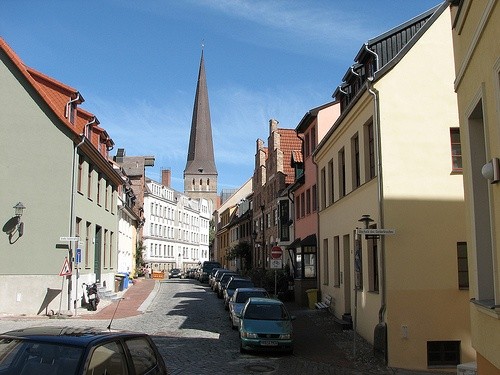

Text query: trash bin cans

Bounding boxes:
[305,289,319,309]
[115,272,129,292]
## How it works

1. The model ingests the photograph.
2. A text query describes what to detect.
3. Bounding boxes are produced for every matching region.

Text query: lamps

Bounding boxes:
[251,230,266,248]
[358,215,380,241]
[13,202,26,236]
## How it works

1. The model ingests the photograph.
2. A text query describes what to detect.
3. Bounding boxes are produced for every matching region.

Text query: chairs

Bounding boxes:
[20,344,61,375]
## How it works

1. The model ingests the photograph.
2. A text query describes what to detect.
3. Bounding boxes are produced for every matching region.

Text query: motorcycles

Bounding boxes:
[82,278,100,312]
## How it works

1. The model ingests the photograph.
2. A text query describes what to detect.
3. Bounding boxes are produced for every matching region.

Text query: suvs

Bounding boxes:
[222,275,256,310]
[184,260,236,293]
[216,272,242,297]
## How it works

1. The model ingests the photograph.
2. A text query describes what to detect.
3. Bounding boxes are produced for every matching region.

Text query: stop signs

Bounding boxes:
[270,246,283,258]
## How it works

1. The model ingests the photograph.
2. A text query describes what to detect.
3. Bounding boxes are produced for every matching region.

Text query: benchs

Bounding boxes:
[314,295,332,315]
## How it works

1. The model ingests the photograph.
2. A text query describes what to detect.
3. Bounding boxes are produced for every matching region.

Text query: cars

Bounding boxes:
[228,287,270,330]
[236,297,297,354]
[0,325,166,375]
[168,268,182,280]
